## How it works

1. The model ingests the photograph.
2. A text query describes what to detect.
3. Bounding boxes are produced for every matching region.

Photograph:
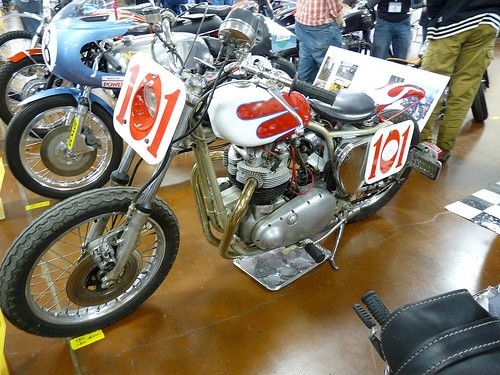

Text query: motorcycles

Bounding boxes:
[0,0,490,339]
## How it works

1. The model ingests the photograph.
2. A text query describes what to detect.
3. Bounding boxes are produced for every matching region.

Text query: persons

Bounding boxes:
[159,0,239,13]
[420,0,500,159]
[361,0,413,65]
[295,0,347,83]
